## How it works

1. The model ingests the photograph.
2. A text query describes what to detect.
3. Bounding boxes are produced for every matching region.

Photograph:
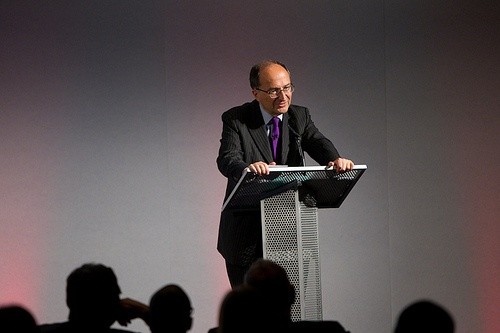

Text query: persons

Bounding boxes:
[0,259,351,333]
[393,299,456,333]
[216,59,354,321]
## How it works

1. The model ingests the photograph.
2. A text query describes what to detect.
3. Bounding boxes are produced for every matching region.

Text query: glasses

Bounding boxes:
[256,83,295,98]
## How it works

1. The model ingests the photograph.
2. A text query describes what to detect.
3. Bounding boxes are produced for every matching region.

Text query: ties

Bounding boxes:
[271,117,281,161]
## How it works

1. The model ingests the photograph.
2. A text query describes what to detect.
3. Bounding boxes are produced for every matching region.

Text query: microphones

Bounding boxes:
[287,124,305,166]
